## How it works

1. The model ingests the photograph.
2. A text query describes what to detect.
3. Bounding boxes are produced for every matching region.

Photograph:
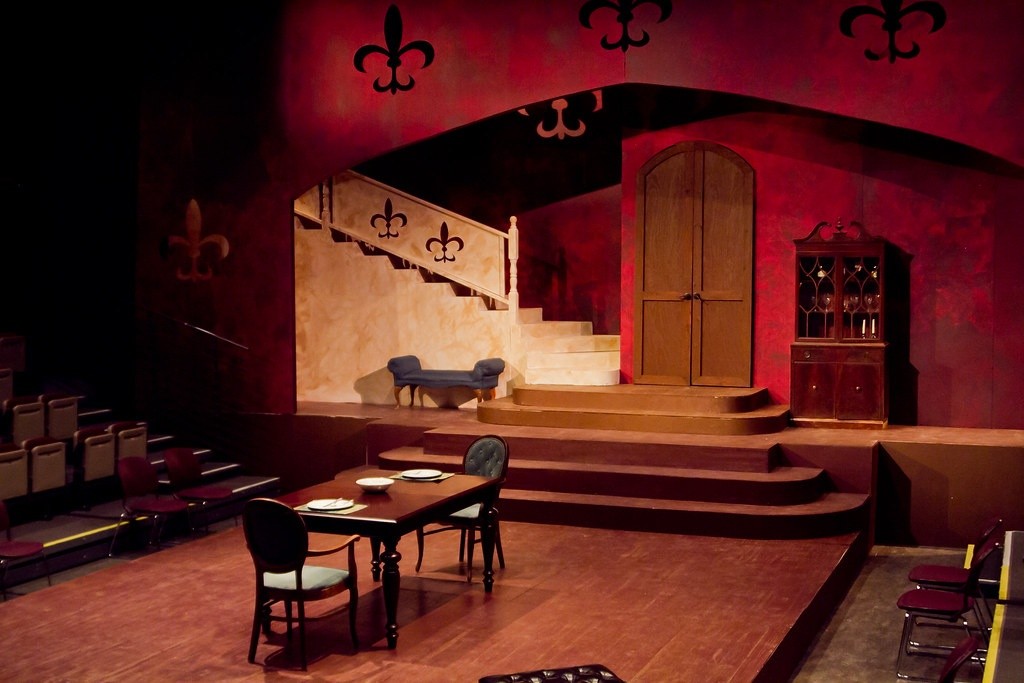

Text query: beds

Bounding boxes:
[73,426,115,482]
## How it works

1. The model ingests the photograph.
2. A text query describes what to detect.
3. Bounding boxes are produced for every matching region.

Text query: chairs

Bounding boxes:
[163,447,238,536]
[20,436,65,494]
[38,391,78,440]
[3,397,44,448]
[108,456,196,557]
[109,420,147,461]
[937,637,978,683]
[416,434,509,584]
[0,368,13,410]
[0,334,24,373]
[0,443,28,500]
[0,501,51,600]
[905,516,1004,662]
[896,538,999,681]
[243,498,360,672]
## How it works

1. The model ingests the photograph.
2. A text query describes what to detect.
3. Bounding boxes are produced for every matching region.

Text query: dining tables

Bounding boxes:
[264,469,501,648]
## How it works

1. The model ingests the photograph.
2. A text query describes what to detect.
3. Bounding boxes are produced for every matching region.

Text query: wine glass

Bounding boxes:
[811,292,880,313]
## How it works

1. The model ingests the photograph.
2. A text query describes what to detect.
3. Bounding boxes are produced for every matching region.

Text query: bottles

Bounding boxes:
[817,265,827,278]
[871,266,878,279]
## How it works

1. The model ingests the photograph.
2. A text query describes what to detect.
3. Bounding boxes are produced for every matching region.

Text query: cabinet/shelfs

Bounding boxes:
[789,216,893,430]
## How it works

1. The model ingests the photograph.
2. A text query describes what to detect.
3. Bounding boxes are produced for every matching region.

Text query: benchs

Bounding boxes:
[387,355,505,409]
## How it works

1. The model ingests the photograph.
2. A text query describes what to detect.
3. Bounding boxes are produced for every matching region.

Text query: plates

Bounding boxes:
[307,499,354,510]
[401,469,442,478]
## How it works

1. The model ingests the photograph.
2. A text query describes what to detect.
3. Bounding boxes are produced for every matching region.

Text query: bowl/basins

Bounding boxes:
[356,477,395,490]
